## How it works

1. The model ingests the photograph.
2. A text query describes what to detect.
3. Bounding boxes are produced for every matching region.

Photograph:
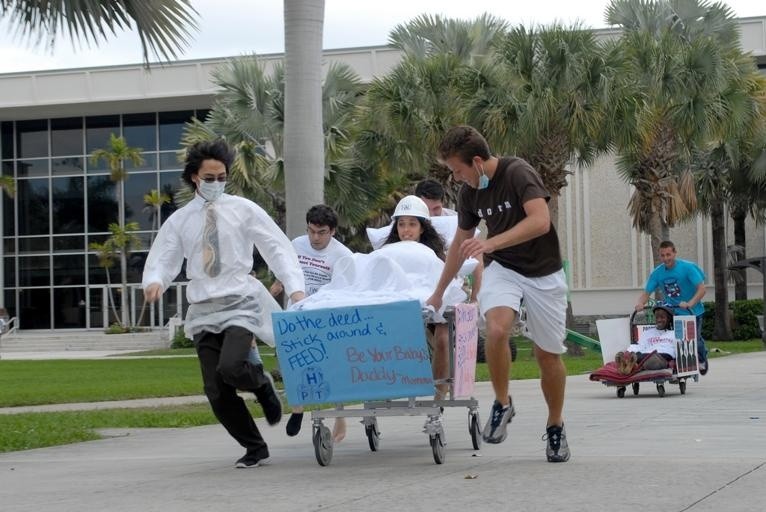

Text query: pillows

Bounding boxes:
[452,257,479,277]
[362,211,482,257]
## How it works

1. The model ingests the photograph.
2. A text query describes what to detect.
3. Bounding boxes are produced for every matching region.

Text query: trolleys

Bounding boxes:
[589,299,706,397]
[266,296,487,465]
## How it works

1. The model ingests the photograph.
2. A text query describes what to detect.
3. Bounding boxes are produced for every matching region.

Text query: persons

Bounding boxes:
[634,240,710,384]
[379,195,446,261]
[142,138,305,467]
[274,204,354,440]
[613,304,676,375]
[425,125,572,462]
[414,181,484,401]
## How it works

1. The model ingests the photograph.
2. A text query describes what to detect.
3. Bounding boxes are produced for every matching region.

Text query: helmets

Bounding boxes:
[391,195,429,220]
[653,301,673,316]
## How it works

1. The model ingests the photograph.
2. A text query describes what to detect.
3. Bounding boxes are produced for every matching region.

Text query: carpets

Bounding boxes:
[588,349,674,386]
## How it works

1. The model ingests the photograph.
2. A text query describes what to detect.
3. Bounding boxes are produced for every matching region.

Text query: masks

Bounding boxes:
[473,161,489,189]
[197,178,226,201]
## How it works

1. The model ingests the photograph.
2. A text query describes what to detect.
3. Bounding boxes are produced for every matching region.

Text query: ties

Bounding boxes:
[202,204,220,277]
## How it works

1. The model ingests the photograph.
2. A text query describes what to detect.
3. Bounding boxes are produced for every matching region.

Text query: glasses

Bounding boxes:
[199,176,227,183]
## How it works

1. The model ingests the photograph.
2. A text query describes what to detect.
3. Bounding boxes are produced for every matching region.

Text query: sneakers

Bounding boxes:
[542,422,570,462]
[255,372,281,424]
[483,396,515,443]
[699,351,708,375]
[235,442,270,468]
